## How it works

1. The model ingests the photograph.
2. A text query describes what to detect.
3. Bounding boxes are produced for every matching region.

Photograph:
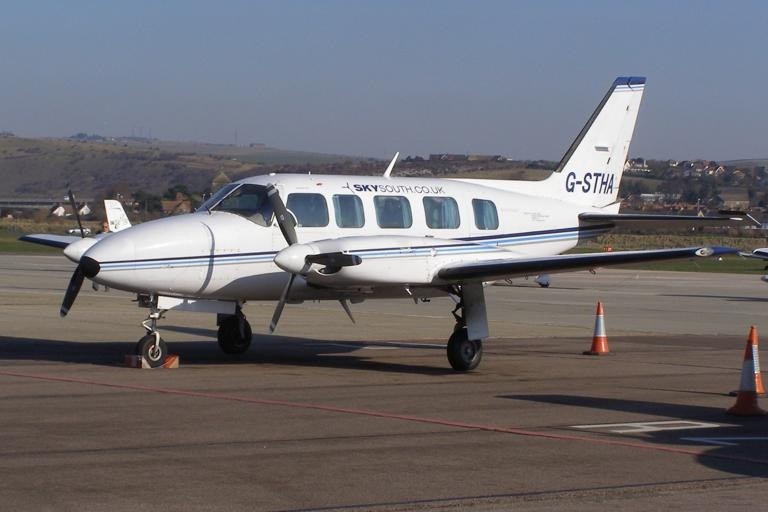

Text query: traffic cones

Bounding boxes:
[582,301,615,358]
[724,324,767,418]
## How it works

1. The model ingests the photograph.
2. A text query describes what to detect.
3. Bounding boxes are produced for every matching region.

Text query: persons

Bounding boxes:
[92,221,113,292]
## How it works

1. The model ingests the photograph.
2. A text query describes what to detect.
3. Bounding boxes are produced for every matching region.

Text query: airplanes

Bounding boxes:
[17,73,763,369]
